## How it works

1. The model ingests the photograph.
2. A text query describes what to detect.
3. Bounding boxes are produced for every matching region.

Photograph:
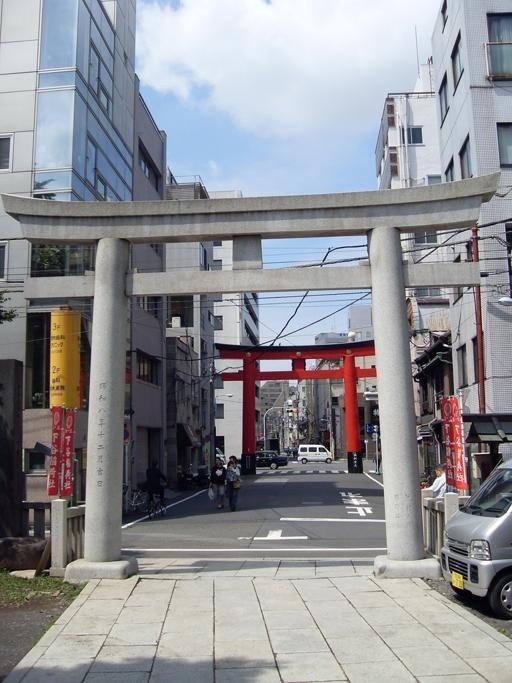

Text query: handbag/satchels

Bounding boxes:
[233,480,240,488]
[208,486,214,500]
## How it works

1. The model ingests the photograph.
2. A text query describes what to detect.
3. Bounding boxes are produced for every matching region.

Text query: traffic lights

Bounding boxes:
[286,409,293,412]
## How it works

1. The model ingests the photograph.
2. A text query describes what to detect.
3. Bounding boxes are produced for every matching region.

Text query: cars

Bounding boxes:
[216,447,226,468]
[256,450,288,468]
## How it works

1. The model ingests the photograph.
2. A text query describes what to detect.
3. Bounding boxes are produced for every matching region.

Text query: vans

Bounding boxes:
[296,443,333,463]
[438,458,511,620]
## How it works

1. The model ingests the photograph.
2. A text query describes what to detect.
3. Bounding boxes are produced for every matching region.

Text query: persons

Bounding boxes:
[226,455,241,511]
[225,460,240,482]
[209,456,228,509]
[146,459,169,506]
[426,462,447,498]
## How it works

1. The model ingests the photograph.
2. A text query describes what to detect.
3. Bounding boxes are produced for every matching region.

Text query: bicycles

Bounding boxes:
[124,483,149,513]
[146,489,167,519]
